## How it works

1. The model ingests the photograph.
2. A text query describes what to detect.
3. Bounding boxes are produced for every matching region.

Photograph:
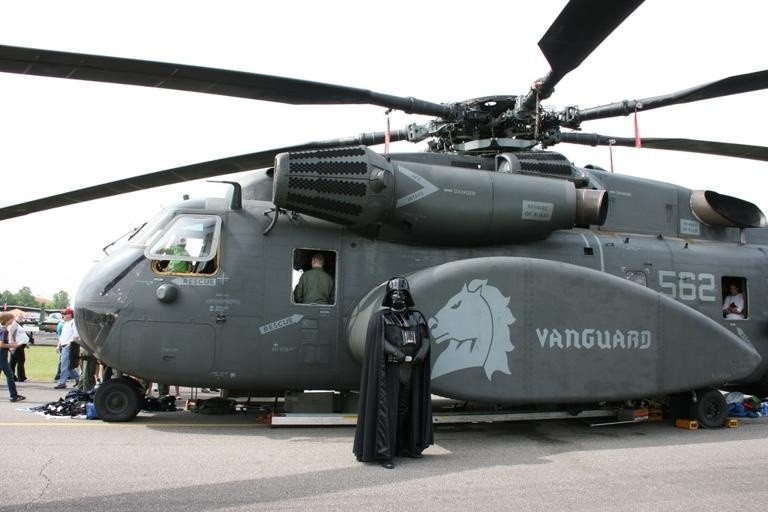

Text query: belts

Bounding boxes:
[61,344,68,348]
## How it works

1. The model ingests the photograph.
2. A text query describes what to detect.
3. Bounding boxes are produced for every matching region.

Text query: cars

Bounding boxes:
[20,308,62,335]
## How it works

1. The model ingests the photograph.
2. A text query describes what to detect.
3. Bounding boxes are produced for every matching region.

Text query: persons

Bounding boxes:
[160,239,192,273]
[722,283,746,320]
[0,307,182,402]
[356,276,431,469]
[294,251,335,305]
[201,387,220,393]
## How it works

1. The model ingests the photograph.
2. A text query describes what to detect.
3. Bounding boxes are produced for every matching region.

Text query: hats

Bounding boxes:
[60,307,75,315]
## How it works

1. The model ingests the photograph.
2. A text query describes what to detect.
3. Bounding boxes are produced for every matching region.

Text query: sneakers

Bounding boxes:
[145,388,220,400]
[14,377,28,382]
[54,383,66,389]
[9,394,26,402]
[73,380,79,387]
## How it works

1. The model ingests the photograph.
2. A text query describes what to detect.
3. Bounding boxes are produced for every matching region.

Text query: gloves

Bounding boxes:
[384,339,406,363]
[412,337,430,363]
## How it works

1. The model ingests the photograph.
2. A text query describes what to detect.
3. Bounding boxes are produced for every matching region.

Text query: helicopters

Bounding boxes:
[0,0,766,430]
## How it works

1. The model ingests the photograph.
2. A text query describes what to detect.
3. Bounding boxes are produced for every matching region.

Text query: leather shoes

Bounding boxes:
[379,461,394,469]
[412,452,424,458]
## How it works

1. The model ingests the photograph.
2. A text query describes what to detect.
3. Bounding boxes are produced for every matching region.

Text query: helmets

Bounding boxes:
[381,277,415,307]
